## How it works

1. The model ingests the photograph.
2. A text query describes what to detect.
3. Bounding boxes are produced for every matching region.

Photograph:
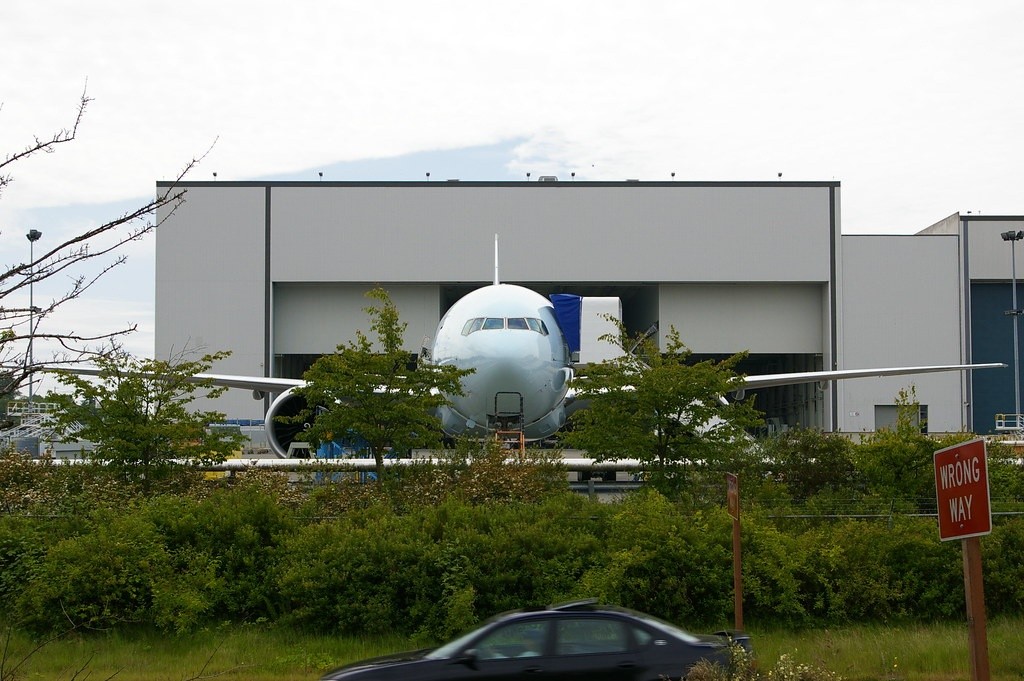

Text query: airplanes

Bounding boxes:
[1,234,1008,459]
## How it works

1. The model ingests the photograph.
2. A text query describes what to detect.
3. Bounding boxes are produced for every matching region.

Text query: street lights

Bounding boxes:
[1002,230,1024,427]
[25,230,42,416]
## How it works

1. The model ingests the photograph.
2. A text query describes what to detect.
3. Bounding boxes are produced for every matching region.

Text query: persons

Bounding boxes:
[485,630,542,659]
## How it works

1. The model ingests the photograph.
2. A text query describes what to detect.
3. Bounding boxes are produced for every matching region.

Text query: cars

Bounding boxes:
[321,600,751,681]
